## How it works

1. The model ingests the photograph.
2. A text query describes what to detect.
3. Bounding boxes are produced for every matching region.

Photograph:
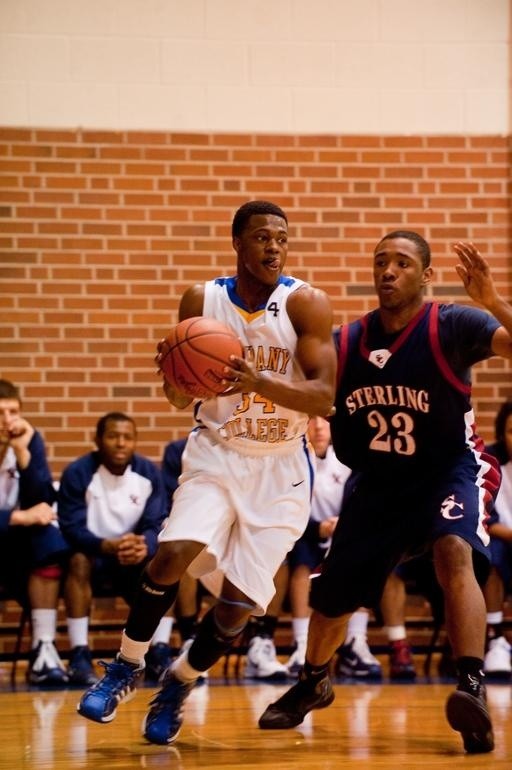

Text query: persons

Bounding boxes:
[259,231,512,752]
[57,411,172,688]
[0,378,71,688]
[155,394,512,685]
[78,199,337,744]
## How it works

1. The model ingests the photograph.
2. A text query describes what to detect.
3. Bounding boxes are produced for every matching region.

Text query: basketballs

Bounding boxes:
[160,316,243,397]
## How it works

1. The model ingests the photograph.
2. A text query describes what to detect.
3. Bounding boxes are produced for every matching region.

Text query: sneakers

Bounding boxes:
[25,638,206,744]
[258,668,335,730]
[244,636,416,681]
[446,682,495,752]
[483,635,511,676]
